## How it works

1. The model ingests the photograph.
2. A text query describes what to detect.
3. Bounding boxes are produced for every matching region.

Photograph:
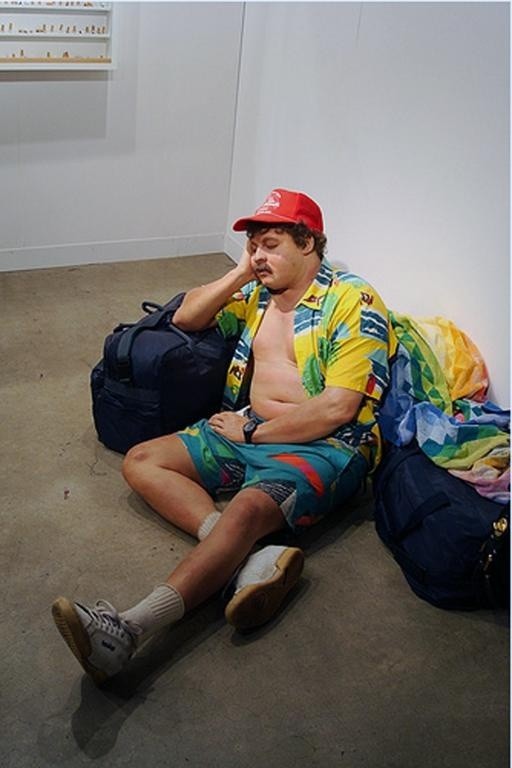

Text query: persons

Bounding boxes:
[49,187,397,694]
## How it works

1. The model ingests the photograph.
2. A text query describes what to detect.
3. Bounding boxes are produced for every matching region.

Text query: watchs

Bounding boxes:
[241,419,259,443]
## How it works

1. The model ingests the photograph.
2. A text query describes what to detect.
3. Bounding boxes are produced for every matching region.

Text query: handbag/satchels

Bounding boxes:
[367,439,508,610]
[90,290,239,458]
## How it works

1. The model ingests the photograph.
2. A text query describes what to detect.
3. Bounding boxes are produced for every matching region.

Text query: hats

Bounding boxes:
[233,189,325,238]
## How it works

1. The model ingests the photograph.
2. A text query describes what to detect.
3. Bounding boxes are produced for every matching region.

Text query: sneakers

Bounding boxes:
[49,596,146,692]
[225,544,304,629]
